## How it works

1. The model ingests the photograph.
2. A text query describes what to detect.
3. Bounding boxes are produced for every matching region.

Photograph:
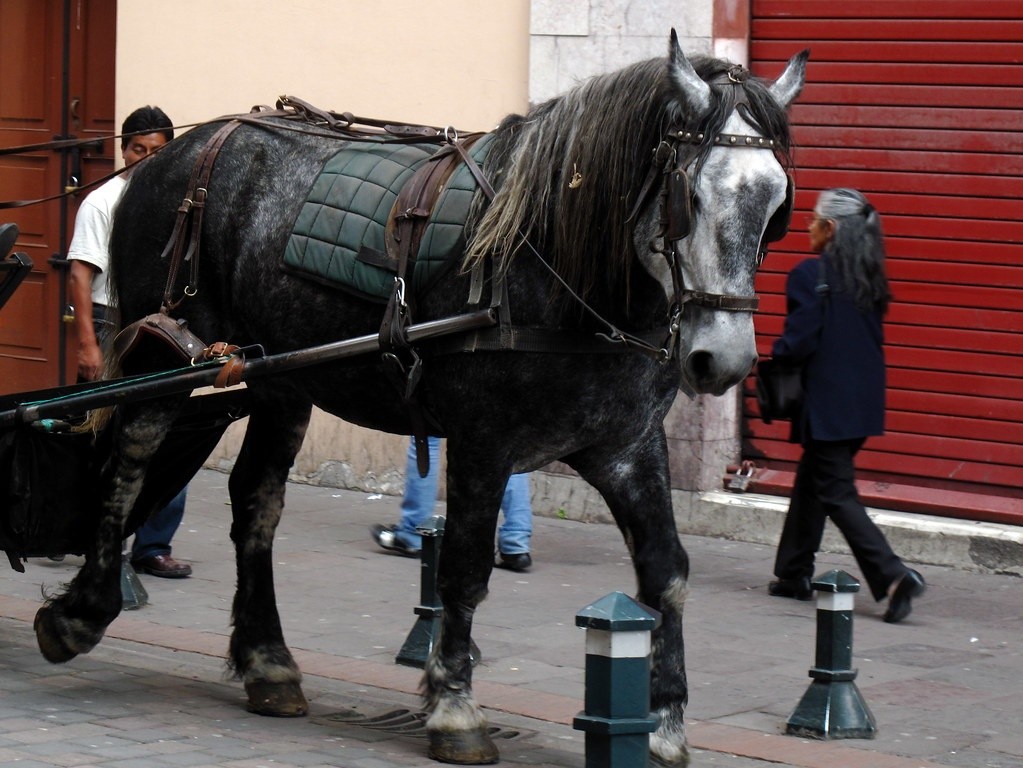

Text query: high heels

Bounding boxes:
[769,576,813,601]
[883,570,926,622]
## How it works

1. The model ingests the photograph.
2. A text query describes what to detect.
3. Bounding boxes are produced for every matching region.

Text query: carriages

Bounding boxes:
[0,27,811,768]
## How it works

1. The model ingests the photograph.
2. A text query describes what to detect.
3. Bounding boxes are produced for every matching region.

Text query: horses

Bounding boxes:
[33,26,813,767]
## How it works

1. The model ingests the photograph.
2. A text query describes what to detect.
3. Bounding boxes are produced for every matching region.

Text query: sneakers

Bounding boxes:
[494,548,532,569]
[370,523,419,558]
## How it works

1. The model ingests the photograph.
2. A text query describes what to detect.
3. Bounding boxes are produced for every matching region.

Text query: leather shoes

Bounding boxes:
[131,554,191,576]
[48,554,65,560]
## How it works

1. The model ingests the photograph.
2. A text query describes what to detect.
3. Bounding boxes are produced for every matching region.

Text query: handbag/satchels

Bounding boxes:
[755,358,802,425]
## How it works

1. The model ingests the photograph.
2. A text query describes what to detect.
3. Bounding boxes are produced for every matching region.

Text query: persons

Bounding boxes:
[67,108,193,578]
[770,188,926,623]
[367,433,533,570]
